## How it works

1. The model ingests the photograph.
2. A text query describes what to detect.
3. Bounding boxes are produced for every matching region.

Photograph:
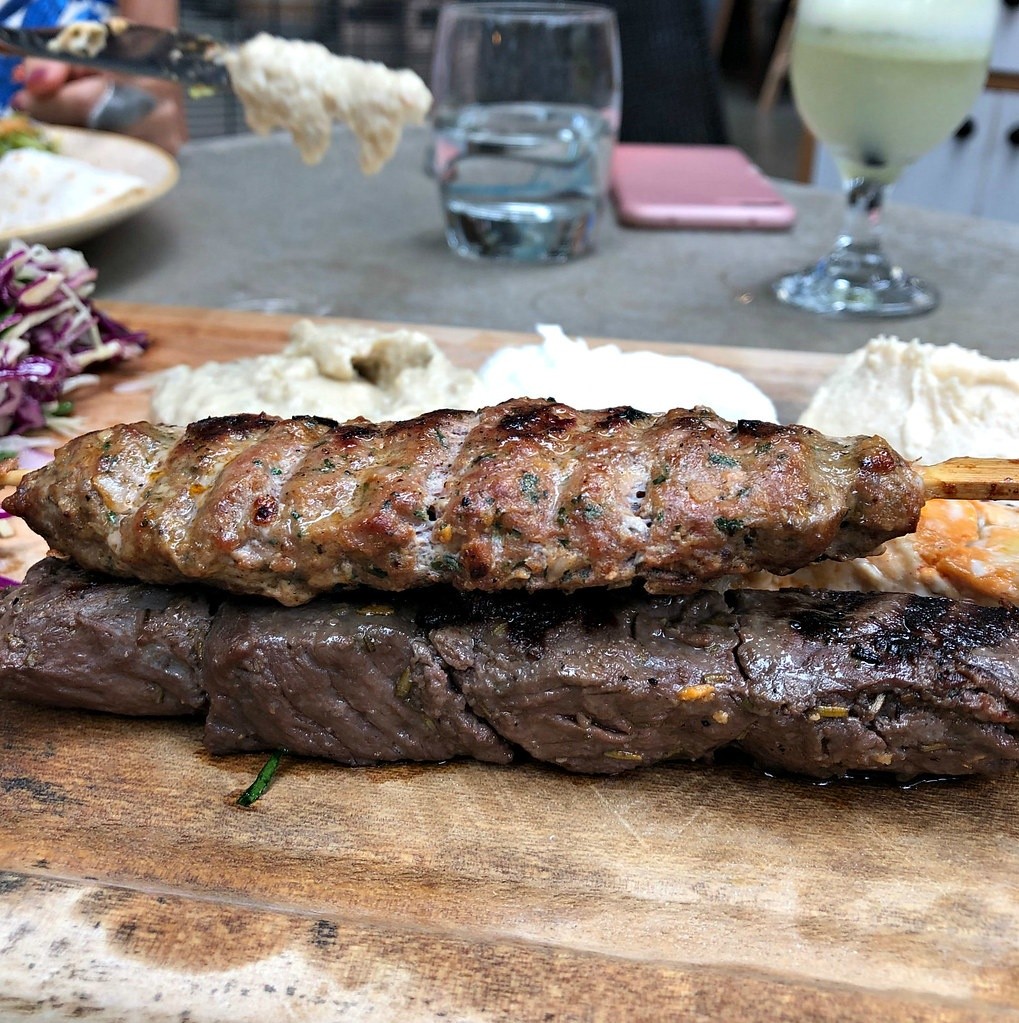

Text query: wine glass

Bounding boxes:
[765,0,1010,311]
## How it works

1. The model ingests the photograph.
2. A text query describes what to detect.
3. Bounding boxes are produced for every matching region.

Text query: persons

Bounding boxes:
[0,0,190,166]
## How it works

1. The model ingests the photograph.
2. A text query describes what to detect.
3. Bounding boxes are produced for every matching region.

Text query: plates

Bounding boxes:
[0,296,1019,1023]
[0,124,178,251]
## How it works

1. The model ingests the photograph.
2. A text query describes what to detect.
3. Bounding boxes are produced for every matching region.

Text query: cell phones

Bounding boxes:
[611,140,796,231]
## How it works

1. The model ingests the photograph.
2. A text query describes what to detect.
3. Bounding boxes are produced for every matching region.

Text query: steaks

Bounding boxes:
[0,555,1019,786]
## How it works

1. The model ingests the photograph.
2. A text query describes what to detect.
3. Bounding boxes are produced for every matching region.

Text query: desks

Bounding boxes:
[76,121,1018,360]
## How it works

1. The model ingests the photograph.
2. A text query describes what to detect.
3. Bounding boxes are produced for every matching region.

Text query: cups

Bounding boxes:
[429,1,627,268]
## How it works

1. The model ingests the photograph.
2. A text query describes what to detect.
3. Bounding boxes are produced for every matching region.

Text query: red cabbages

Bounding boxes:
[1,243,154,466]
